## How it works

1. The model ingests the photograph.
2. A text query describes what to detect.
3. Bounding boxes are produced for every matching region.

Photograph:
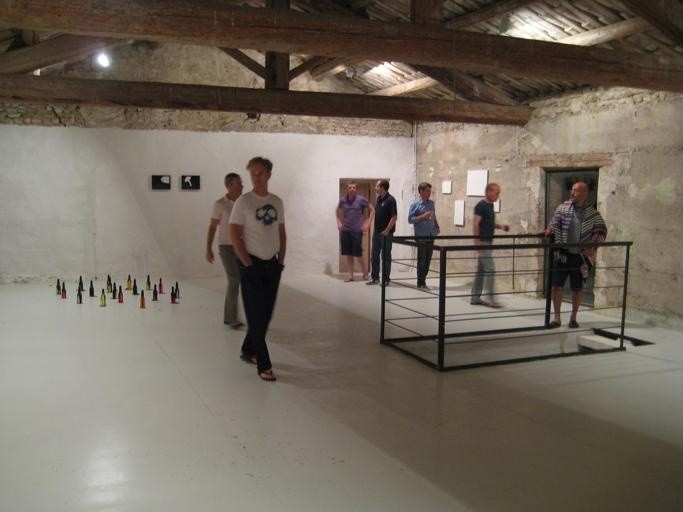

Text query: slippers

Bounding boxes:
[239,355,256,364]
[257,368,276,381]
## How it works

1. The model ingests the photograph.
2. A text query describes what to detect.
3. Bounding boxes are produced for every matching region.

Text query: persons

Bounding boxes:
[408,182,440,289]
[336,181,374,283]
[471,183,510,307]
[545,182,607,327]
[206,173,245,327]
[367,180,397,286]
[229,156,286,380]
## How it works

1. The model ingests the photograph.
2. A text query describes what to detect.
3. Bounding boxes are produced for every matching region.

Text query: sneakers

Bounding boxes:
[381,280,390,285]
[345,278,353,281]
[366,280,379,284]
[569,320,578,328]
[489,302,503,307]
[224,321,242,328]
[550,320,561,328]
[363,273,368,280]
[471,301,488,305]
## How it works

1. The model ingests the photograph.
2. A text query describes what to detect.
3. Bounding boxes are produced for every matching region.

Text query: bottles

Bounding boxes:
[170,286,176,304]
[76,275,83,304]
[175,281,180,298]
[106,274,112,293]
[100,288,107,307]
[133,278,138,295]
[145,274,152,290]
[126,274,132,290]
[158,278,163,294]
[112,282,118,299]
[152,284,158,301]
[55,278,66,299]
[89,280,94,297]
[139,289,146,308]
[118,285,124,303]
